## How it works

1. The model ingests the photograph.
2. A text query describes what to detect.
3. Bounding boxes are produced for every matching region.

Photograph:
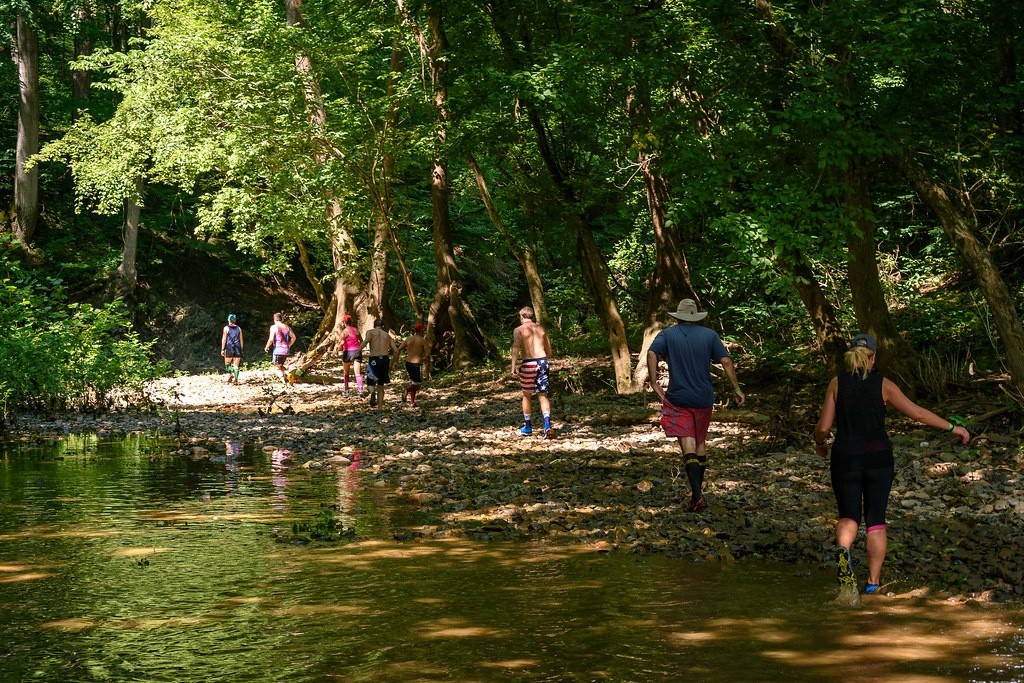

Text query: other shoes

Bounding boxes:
[234,381,238,385]
[359,392,365,398]
[286,374,294,385]
[228,374,234,382]
[402,388,408,402]
[344,390,349,398]
[370,391,376,407]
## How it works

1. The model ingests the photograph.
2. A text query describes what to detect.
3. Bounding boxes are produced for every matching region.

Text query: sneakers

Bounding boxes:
[543,426,554,439]
[687,493,708,513]
[520,427,533,436]
[863,580,882,594]
[835,546,855,586]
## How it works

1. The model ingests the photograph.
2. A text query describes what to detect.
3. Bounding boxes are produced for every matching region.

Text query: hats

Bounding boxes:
[342,315,353,324]
[667,298,707,322]
[851,334,876,352]
[228,314,236,322]
[415,323,423,332]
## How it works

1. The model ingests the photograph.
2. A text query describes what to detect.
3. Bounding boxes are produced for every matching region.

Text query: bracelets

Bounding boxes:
[816,441,824,445]
[734,389,740,392]
[222,349,224,351]
[948,423,954,431]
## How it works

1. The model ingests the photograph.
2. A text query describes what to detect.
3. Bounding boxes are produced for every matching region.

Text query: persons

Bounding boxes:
[265,313,296,389]
[816,334,970,596]
[391,324,430,408]
[511,307,555,440]
[330,315,366,399]
[221,314,243,386]
[647,299,745,512]
[355,319,399,414]
